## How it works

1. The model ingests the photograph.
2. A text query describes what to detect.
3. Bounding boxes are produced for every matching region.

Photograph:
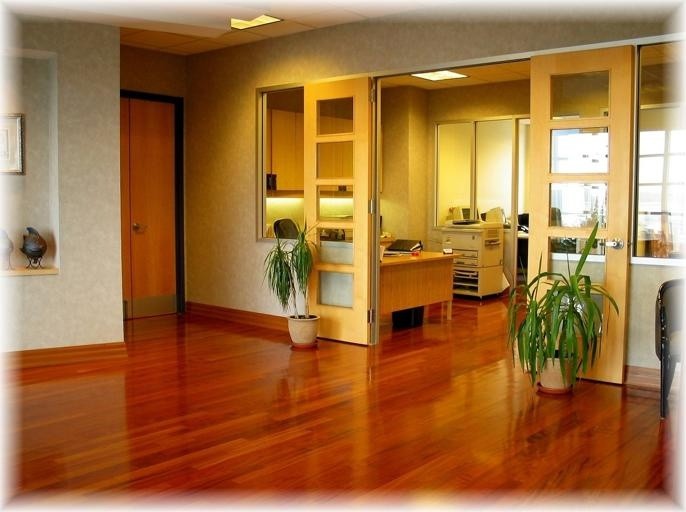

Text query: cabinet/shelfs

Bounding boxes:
[266,108,382,198]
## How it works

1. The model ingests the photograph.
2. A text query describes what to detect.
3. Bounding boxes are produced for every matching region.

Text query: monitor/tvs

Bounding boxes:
[453,206,480,222]
[481,207,503,223]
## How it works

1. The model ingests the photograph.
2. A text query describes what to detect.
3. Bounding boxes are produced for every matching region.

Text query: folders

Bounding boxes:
[386,240,423,252]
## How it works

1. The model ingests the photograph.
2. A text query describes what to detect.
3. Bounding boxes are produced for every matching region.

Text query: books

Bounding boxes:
[387,240,421,250]
[410,244,423,252]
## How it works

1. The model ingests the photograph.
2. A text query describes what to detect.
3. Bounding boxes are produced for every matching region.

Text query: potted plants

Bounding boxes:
[260,215,322,348]
[504,221,619,395]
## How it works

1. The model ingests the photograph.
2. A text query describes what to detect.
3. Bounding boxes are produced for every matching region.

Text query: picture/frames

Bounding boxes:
[0,113,27,176]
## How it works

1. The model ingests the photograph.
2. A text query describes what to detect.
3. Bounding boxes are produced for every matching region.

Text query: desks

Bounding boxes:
[381,252,463,321]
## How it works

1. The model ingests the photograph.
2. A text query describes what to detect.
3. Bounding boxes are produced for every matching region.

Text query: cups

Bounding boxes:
[379,245,384,262]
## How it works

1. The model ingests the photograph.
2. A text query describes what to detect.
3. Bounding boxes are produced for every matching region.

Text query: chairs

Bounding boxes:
[274,219,299,239]
[655,279,686,419]
